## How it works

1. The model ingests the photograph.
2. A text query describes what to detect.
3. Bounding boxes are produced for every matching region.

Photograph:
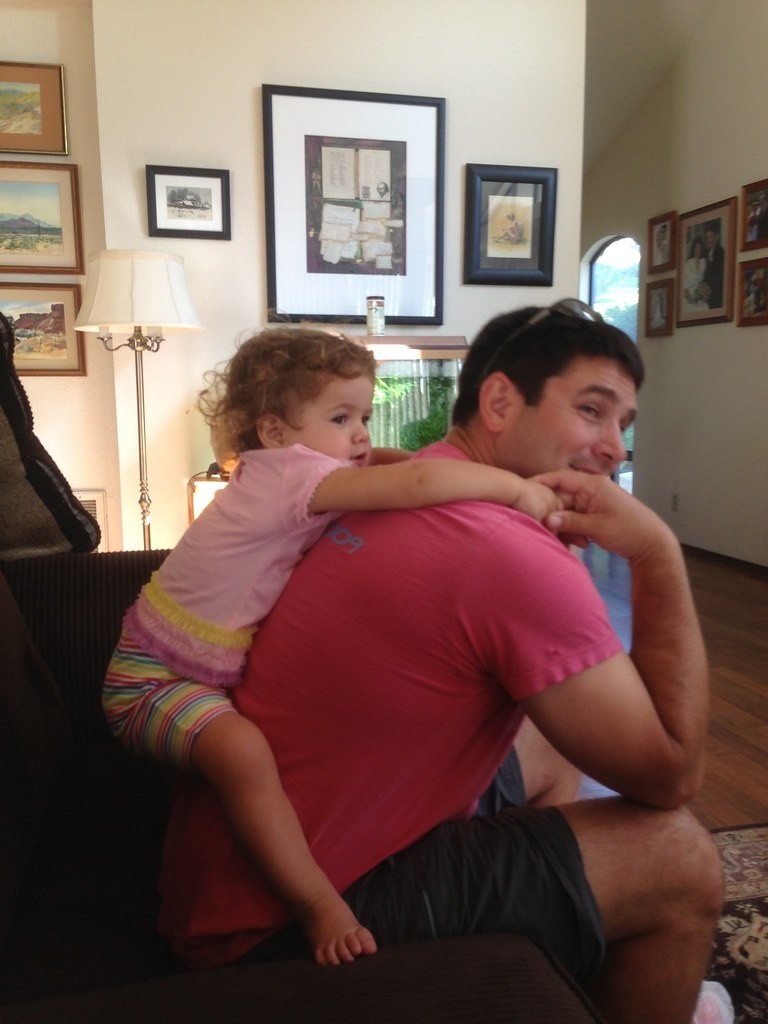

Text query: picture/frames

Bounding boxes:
[144,164,232,241]
[0,160,86,275]
[674,193,739,327]
[0,60,72,156]
[644,277,673,338]
[736,256,768,328]
[738,178,768,253]
[261,84,447,326]
[647,210,675,272]
[464,162,557,287]
[0,280,88,376]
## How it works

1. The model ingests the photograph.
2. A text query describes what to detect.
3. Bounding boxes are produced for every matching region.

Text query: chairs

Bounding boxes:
[1,349,603,1024]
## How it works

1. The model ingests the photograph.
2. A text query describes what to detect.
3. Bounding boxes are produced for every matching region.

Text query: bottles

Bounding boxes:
[367,295,386,336]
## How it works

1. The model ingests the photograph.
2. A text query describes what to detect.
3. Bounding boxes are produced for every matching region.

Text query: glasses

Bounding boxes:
[478,298,603,384]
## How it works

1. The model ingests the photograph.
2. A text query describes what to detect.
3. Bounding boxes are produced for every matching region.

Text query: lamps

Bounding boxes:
[78,246,209,554]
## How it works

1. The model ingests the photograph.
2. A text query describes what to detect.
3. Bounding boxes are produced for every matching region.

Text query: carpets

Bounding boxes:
[709,822,768,1024]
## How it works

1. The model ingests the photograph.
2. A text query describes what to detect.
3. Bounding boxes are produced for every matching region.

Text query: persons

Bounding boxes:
[160,297,726,1024]
[649,192,768,322]
[102,329,565,966]
